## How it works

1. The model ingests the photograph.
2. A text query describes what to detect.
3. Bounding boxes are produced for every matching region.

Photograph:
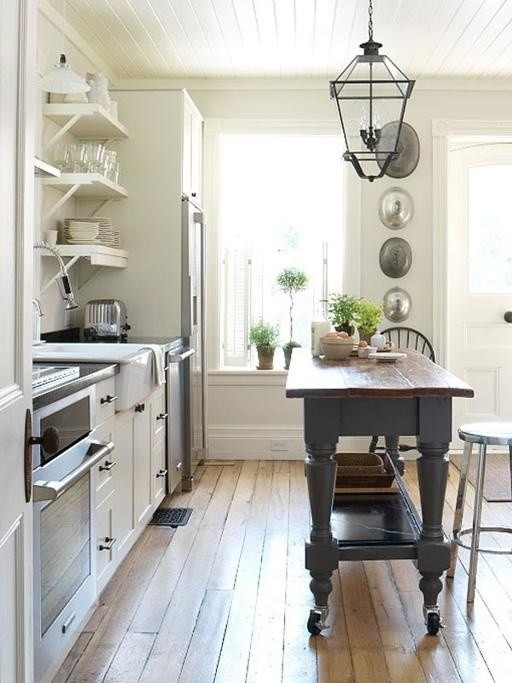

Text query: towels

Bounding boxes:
[139,343,167,385]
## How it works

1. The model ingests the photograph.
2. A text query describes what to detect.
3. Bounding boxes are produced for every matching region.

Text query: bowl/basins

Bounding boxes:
[357,346,377,358]
[319,337,353,360]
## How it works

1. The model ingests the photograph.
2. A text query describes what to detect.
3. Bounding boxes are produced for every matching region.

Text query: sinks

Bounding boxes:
[32,342,166,413]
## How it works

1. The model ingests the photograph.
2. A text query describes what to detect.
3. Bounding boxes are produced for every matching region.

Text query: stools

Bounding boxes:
[447,420,512,601]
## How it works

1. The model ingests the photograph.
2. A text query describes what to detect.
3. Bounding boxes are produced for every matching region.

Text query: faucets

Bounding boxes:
[33,297,45,318]
[35,242,79,312]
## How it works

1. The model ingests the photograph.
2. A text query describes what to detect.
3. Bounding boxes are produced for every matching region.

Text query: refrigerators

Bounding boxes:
[180,196,211,490]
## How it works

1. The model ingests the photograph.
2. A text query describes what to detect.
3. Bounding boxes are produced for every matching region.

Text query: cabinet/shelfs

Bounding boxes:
[88,371,120,606]
[109,374,153,583]
[78,86,204,337]
[38,52,132,269]
[149,371,173,521]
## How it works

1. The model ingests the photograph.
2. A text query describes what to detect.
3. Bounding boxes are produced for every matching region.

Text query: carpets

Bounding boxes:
[447,452,512,501]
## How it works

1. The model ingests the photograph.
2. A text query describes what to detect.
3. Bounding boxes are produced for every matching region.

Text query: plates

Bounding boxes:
[64,215,120,248]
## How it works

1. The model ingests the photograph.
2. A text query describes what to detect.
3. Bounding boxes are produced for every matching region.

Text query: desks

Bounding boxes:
[287,343,475,636]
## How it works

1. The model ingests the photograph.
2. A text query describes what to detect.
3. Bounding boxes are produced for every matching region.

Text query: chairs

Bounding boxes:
[367,326,436,454]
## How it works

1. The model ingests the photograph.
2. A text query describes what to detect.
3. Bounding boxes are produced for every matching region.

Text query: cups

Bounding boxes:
[52,142,120,183]
[46,229,59,245]
[110,100,118,120]
[311,320,331,357]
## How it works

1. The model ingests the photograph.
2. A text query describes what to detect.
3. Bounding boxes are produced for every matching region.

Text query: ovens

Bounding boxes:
[26,371,121,682]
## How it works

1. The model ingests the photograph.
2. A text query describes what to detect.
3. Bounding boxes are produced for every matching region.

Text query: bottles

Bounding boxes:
[370,331,386,351]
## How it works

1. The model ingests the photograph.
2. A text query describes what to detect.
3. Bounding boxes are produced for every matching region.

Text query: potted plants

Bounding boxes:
[325,291,355,336]
[249,318,281,369]
[276,267,308,369]
[354,299,381,343]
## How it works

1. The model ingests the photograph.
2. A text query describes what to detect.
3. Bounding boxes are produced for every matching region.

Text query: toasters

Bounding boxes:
[84,299,130,338]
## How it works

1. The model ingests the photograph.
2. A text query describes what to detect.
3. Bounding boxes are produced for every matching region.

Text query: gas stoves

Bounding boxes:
[32,362,118,396]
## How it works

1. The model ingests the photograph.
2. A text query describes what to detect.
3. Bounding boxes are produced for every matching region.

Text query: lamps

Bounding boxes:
[326,0,419,182]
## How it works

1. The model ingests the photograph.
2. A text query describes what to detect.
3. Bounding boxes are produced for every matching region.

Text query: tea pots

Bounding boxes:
[85,74,111,110]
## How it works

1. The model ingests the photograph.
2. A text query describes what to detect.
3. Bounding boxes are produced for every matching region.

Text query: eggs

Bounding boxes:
[359,340,367,347]
[326,333,337,337]
[338,332,348,337]
